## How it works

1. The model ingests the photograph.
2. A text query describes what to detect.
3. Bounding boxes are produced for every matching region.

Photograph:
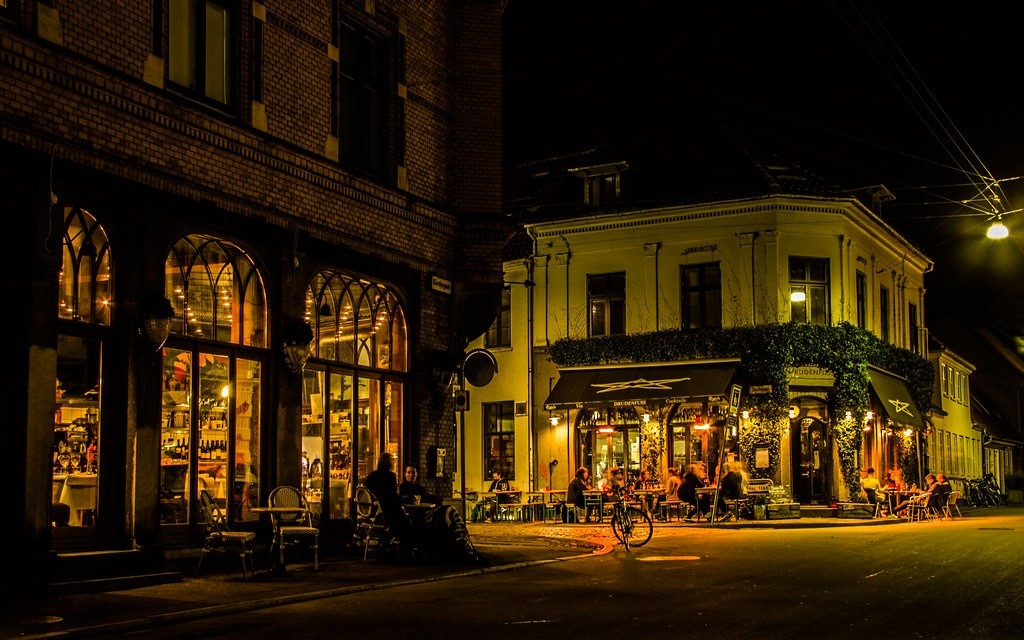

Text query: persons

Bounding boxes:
[647,467,682,521]
[863,468,901,519]
[633,471,654,522]
[302,451,322,478]
[677,461,714,523]
[893,473,951,518]
[487,459,500,478]
[363,453,417,562]
[593,468,624,523]
[711,461,753,522]
[466,469,510,523]
[566,467,603,524]
[400,463,444,548]
[881,472,896,488]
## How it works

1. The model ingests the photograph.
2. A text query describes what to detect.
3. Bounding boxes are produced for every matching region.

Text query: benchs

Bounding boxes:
[461,478,752,522]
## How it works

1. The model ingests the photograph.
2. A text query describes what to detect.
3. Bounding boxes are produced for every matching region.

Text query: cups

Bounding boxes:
[641,484,663,489]
[414,495,421,503]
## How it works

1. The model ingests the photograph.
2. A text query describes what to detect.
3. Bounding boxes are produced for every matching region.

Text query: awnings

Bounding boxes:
[542,360,738,411]
[866,368,923,428]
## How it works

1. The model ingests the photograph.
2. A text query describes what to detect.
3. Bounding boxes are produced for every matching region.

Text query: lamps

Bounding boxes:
[53,229,98,258]
[846,409,853,419]
[641,406,657,423]
[742,402,752,418]
[312,292,333,318]
[882,428,892,435]
[549,413,564,426]
[787,403,795,418]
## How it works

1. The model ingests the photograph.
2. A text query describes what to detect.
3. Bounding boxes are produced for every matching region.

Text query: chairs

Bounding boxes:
[351,485,393,560]
[193,490,255,583]
[263,486,320,571]
[864,487,962,523]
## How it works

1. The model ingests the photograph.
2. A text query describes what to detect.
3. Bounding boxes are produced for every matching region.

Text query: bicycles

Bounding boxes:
[609,479,653,553]
[959,472,1010,508]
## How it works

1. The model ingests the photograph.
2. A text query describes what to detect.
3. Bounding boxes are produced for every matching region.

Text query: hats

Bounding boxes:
[611,468,624,474]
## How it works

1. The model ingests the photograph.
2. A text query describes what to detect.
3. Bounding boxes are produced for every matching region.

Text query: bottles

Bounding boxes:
[162,437,227,460]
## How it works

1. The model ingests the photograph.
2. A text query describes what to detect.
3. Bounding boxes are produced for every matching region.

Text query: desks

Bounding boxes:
[525,489,568,522]
[462,491,479,522]
[632,487,666,522]
[398,504,439,560]
[694,485,722,521]
[880,489,916,523]
[248,506,309,575]
[53,474,98,527]
[484,490,520,522]
[581,489,613,522]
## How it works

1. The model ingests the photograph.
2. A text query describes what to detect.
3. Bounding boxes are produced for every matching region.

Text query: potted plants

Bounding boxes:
[138,290,177,352]
[281,316,313,375]
[427,348,454,394]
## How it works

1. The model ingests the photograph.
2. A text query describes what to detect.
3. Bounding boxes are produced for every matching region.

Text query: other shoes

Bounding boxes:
[720,512,734,521]
[683,517,695,522]
[887,514,898,519]
[704,512,712,519]
